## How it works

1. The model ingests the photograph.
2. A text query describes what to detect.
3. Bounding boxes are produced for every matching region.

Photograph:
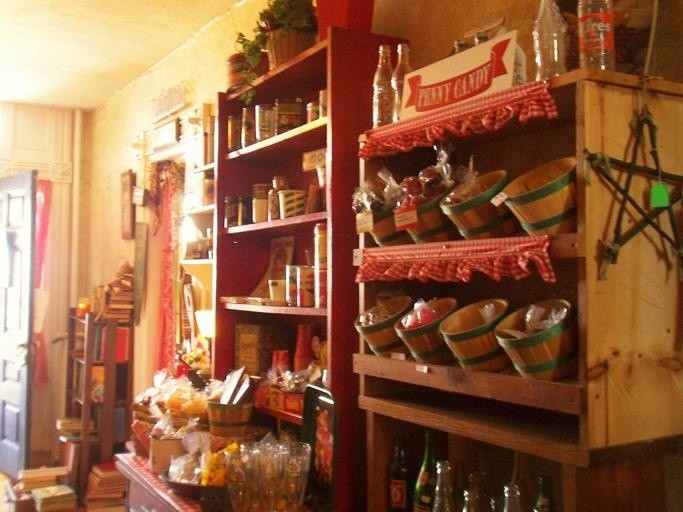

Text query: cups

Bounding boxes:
[148,435,189,474]
[268,281,286,302]
[271,322,311,377]
[279,189,303,218]
[229,441,314,511]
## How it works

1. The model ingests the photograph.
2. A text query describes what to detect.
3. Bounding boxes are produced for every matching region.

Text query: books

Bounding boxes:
[5,270,134,512]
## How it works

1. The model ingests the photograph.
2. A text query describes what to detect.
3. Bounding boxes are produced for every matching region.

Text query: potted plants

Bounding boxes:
[230,0,321,106]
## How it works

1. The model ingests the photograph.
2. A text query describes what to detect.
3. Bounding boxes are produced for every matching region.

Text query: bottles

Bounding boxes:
[197,226,213,259]
[304,89,327,121]
[373,43,408,126]
[531,0,575,83]
[385,427,562,511]
[266,174,292,225]
[577,0,619,71]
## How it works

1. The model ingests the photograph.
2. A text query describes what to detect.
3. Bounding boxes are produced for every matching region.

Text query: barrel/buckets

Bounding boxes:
[395,298,456,367]
[440,169,508,239]
[394,178,455,243]
[503,155,576,237]
[354,295,413,358]
[494,299,572,380]
[365,207,404,245]
[439,299,508,373]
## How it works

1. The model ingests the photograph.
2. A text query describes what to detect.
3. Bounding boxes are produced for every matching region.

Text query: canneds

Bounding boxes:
[228,114,241,151]
[284,223,327,309]
[251,183,271,223]
[254,104,273,139]
[240,107,255,146]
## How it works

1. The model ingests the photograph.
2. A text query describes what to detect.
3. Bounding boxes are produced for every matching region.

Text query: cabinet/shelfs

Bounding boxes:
[212,28,412,511]
[179,160,215,311]
[62,305,134,502]
[113,452,205,511]
[354,67,682,512]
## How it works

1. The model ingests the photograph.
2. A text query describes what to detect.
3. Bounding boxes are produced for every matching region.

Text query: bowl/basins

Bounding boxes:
[158,468,225,498]
